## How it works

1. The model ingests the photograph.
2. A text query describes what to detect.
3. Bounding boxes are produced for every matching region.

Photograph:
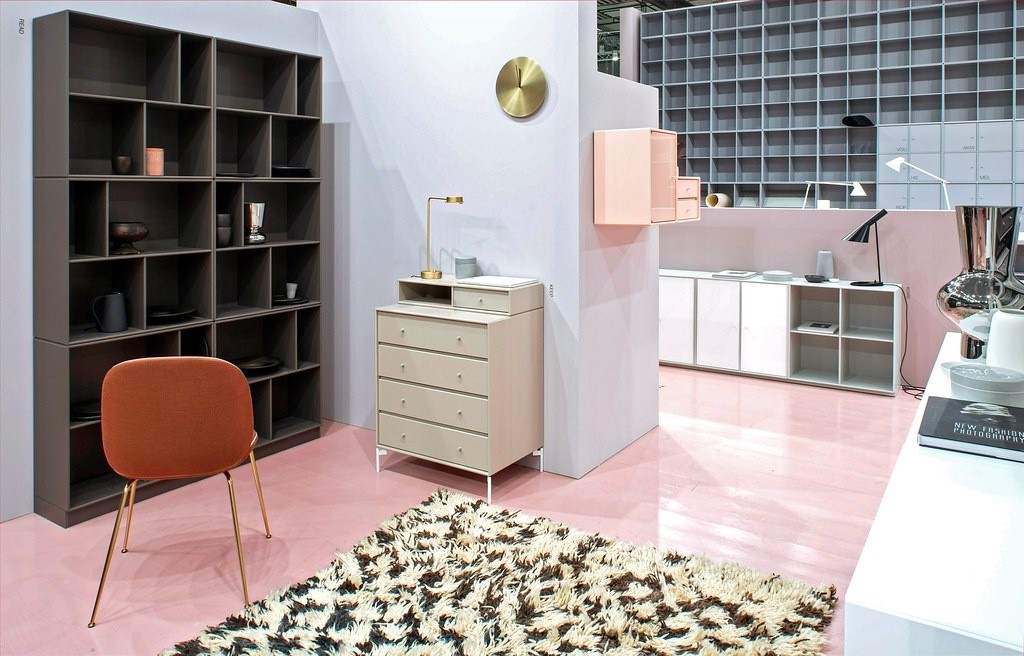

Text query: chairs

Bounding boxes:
[88,357,273,628]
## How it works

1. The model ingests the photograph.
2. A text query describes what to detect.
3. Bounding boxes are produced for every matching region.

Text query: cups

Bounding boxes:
[286,283,297,298]
[111,156,132,174]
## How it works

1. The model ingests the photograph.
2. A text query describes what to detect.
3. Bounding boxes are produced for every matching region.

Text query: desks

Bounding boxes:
[843,333,1024,656]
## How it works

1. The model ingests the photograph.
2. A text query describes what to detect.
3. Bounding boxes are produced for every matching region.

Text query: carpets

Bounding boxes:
[159,488,838,656]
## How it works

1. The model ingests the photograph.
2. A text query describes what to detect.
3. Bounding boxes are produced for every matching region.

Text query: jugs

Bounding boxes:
[92,291,127,332]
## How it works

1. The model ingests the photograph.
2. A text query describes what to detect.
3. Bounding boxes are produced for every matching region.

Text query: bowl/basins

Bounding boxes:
[805,275,825,282]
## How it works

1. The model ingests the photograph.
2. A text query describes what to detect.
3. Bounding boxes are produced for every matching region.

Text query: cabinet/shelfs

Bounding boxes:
[33,10,324,529]
[375,275,545,504]
[593,128,702,225]
[639,0,1024,209]
[659,269,902,397]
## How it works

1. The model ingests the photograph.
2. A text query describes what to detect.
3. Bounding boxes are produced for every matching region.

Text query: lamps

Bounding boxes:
[842,209,888,286]
[421,197,463,280]
[802,180,867,209]
[885,157,951,210]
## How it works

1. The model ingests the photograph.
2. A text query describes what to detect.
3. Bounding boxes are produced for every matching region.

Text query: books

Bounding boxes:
[712,270,757,280]
[457,274,540,287]
